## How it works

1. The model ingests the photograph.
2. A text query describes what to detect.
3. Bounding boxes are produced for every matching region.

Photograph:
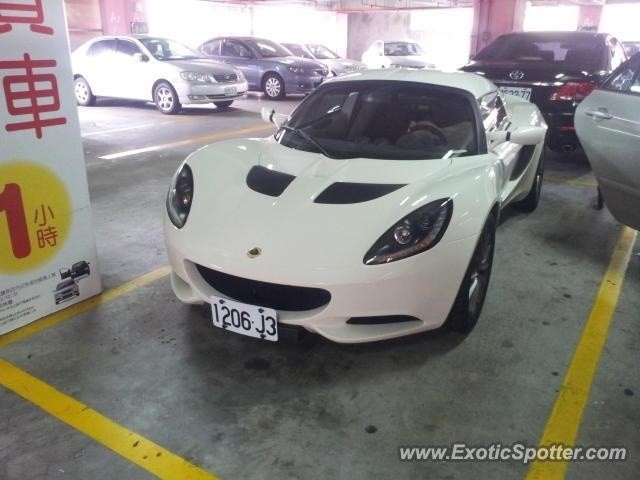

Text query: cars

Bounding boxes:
[457,31,630,158]
[71,33,250,115]
[188,34,367,101]
[361,38,439,72]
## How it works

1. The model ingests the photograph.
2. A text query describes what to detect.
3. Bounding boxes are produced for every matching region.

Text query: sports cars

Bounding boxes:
[161,67,548,348]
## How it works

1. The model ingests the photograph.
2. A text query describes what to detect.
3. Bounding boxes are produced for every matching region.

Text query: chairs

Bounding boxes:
[347,102,475,151]
[146,43,157,55]
